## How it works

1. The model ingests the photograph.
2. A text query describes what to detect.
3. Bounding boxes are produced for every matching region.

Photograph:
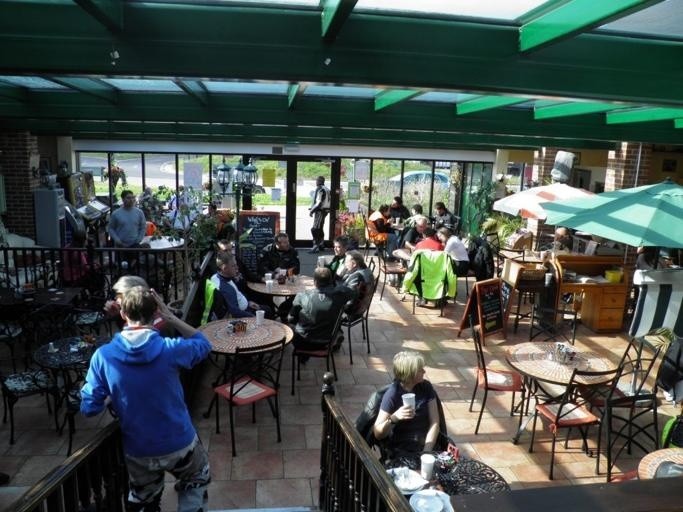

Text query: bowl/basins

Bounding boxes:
[604,270,624,282]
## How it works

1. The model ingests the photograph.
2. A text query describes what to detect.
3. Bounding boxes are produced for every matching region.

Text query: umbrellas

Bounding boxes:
[492,182,598,224]
[539,177,682,251]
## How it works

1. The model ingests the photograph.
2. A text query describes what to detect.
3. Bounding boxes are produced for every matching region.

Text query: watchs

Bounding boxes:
[387,417,396,426]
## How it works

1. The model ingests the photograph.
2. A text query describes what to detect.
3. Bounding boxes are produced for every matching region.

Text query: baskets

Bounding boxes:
[519,263,548,281]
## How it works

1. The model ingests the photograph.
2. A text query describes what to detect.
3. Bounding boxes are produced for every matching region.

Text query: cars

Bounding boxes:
[390,171,451,189]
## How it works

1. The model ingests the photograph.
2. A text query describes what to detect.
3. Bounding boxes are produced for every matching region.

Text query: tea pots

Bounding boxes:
[434,452,458,473]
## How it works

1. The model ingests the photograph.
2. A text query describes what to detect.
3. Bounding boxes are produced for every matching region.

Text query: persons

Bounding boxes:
[210,251,271,321]
[104,276,176,333]
[480,216,683,270]
[257,233,300,320]
[308,175,331,252]
[108,190,145,266]
[373,350,443,467]
[63,230,101,282]
[78,284,214,511]
[367,197,469,307]
[285,236,375,365]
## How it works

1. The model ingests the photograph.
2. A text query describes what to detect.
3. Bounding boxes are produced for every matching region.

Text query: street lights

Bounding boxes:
[214,158,262,274]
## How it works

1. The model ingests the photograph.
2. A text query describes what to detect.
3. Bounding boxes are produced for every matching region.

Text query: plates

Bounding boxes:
[385,468,454,512]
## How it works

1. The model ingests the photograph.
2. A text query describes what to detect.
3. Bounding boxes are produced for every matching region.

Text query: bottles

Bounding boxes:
[546,341,576,367]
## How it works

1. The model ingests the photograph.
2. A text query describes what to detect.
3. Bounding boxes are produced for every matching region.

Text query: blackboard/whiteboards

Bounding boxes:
[236,209,280,282]
[476,279,506,337]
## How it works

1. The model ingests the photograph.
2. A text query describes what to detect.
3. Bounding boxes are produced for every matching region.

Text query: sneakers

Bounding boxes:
[307,241,324,253]
[331,332,344,352]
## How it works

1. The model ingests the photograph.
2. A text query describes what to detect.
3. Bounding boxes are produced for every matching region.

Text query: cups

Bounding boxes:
[395,218,400,225]
[420,452,434,480]
[401,393,415,412]
[264,267,286,291]
[544,273,552,284]
[255,309,264,327]
[539,252,546,260]
[318,256,325,268]
